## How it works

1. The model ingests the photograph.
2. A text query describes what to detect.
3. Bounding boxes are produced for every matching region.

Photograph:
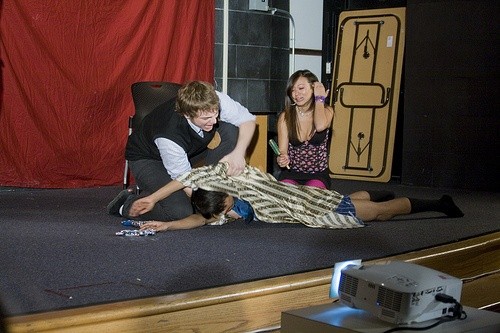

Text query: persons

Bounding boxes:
[107,79,256,223]
[275,69,335,190]
[129,162,464,232]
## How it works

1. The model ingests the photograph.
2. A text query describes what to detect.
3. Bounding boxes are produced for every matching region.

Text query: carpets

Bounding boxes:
[0,177,500,318]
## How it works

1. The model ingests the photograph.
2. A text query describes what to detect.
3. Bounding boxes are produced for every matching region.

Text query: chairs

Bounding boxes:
[123,81,183,191]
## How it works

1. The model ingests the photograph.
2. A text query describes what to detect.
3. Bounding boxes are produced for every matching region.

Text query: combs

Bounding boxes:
[269,139,290,170]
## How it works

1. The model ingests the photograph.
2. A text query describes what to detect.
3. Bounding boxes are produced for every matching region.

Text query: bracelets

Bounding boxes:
[315,95,325,104]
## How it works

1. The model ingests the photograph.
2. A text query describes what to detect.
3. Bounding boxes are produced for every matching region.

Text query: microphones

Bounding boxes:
[293,102,297,105]
[269,139,290,169]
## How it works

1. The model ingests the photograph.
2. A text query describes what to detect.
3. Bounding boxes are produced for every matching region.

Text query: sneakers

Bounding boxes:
[105,189,133,216]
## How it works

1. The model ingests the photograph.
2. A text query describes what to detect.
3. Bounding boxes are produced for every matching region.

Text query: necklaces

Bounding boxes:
[295,106,313,115]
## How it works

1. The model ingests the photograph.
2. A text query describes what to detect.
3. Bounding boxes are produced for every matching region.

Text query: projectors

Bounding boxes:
[338,259,463,326]
